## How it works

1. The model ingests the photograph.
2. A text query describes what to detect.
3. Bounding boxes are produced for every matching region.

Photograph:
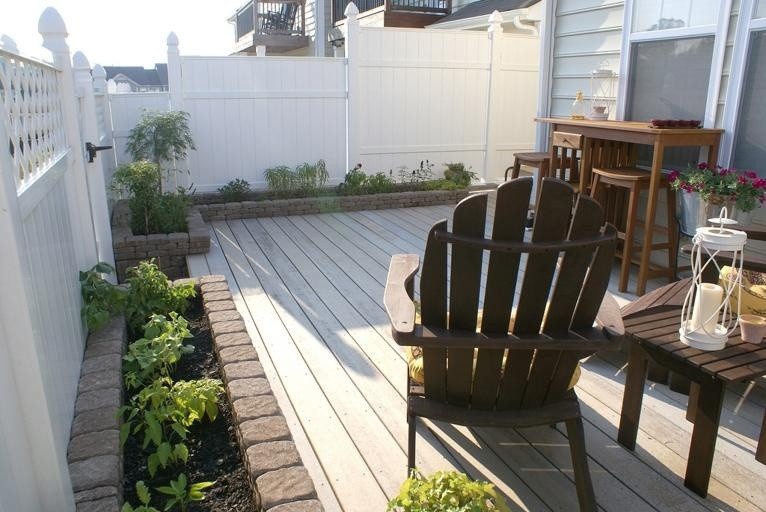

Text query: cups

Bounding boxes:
[738,314,765,343]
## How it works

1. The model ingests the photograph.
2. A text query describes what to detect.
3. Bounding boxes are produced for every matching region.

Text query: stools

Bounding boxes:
[589,165,677,294]
[511,151,571,212]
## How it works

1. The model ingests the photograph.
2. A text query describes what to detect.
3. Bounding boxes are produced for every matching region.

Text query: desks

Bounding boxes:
[535,116,726,297]
[604,277,765,500]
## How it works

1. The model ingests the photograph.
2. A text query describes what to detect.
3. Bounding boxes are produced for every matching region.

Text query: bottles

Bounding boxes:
[570,90,584,119]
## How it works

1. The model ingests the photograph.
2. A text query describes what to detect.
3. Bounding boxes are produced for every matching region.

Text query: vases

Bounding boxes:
[707,194,732,206]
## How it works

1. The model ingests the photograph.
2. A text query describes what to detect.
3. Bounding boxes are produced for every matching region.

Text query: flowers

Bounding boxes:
[669,162,765,213]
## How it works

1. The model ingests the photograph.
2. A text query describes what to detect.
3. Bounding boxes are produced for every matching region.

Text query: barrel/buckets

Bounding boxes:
[678,188,739,237]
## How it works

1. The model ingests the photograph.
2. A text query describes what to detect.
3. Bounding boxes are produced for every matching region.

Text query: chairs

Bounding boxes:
[383,176,617,512]
